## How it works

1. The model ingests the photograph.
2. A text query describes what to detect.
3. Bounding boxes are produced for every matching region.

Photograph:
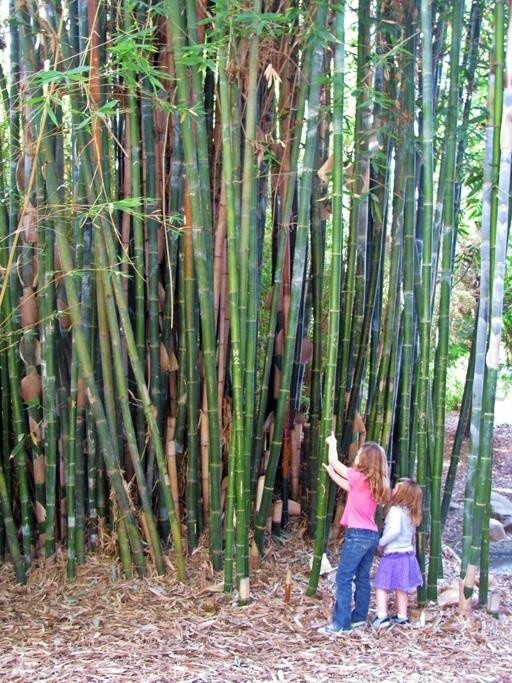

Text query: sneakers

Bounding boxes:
[392,614,409,624]
[317,621,368,635]
[369,616,391,628]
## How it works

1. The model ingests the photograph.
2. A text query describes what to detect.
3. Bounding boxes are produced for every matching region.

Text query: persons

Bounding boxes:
[317,428,391,634]
[371,475,424,631]
